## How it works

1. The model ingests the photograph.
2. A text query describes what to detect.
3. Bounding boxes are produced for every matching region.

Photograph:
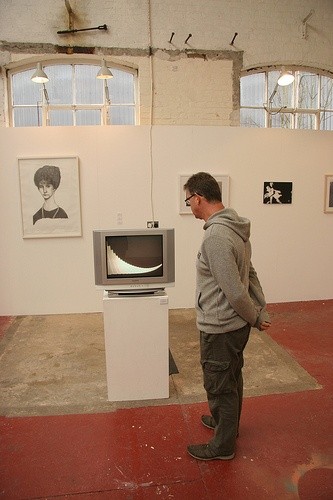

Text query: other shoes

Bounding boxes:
[201,415,216,429]
[186,444,235,460]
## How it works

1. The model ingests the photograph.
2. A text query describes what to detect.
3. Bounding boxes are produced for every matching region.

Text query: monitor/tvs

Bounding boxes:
[93,228,175,293]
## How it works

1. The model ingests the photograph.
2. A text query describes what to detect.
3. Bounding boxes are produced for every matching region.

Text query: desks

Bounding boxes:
[102,289,168,401]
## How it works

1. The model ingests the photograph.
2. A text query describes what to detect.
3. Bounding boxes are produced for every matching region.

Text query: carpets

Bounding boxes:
[0,308,325,417]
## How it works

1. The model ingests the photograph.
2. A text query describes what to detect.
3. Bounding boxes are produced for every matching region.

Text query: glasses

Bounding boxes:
[185,193,203,205]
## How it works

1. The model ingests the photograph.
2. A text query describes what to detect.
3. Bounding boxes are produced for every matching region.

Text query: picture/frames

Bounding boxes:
[323,174,333,214]
[177,174,230,215]
[17,156,82,240]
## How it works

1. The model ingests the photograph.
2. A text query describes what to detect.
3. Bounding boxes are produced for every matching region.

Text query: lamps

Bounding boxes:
[96,57,113,80]
[277,65,294,87]
[30,62,48,83]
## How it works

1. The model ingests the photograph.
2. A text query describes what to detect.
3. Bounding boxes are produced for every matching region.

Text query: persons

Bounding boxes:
[183,172,272,460]
